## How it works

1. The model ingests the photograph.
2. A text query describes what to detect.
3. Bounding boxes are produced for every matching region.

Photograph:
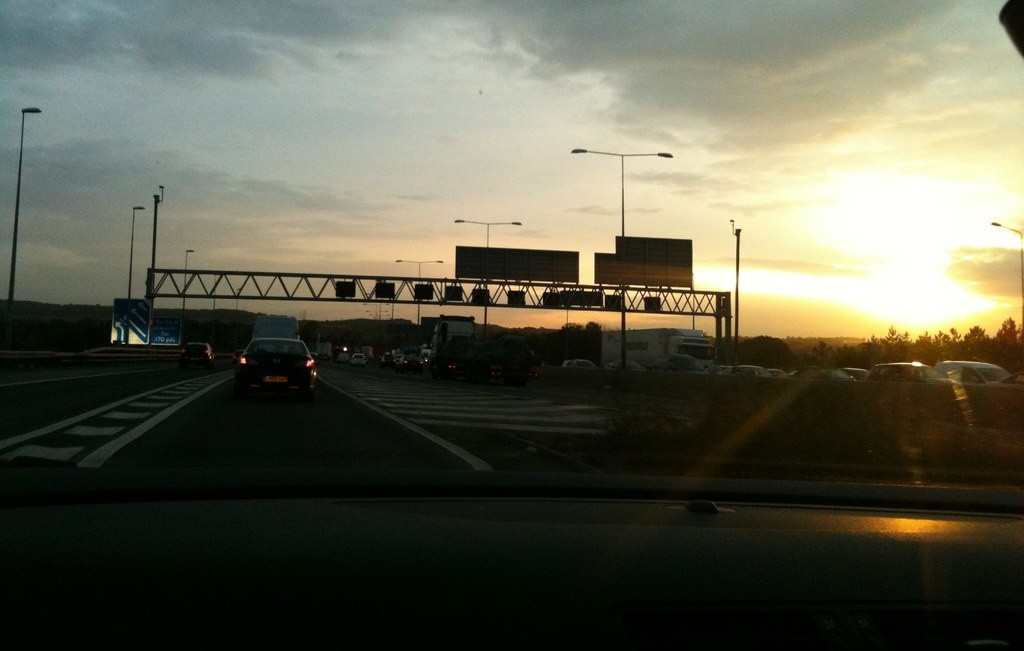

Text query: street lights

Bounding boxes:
[454,219,522,341]
[123,206,146,348]
[5,107,42,353]
[571,145,674,366]
[152,185,164,272]
[179,250,194,350]
[734,227,744,358]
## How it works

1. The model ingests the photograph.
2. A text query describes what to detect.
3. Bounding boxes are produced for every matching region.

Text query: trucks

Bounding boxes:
[252,317,300,342]
[649,331,714,371]
[317,342,332,360]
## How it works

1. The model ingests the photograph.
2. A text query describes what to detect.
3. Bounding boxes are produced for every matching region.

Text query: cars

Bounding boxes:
[231,350,245,364]
[607,358,641,372]
[714,357,1023,395]
[563,360,595,370]
[236,336,319,398]
[350,354,369,367]
[338,331,541,387]
[179,342,215,368]
